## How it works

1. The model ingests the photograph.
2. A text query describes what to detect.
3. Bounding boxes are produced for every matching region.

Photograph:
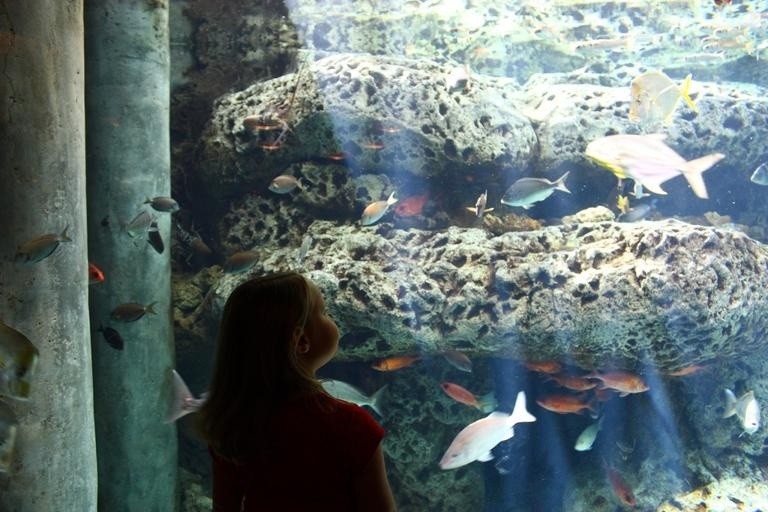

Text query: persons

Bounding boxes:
[199,273,398,511]
